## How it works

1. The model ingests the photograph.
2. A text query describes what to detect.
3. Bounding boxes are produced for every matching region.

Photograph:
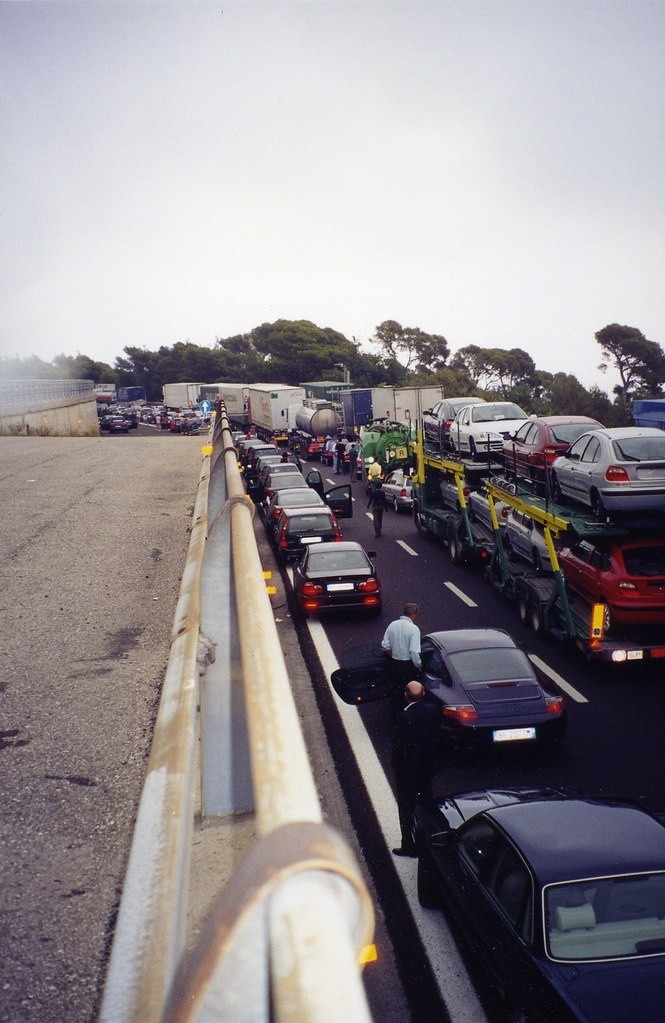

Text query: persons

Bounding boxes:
[181,415,188,435]
[235,442,243,459]
[281,451,293,463]
[349,444,358,482]
[195,396,200,408]
[270,436,277,447]
[136,410,141,424]
[164,404,168,417]
[382,602,424,683]
[245,430,250,440]
[142,409,148,424]
[366,481,388,540]
[179,406,184,412]
[332,437,347,475]
[155,414,161,429]
[242,446,253,464]
[389,679,450,859]
[249,427,254,435]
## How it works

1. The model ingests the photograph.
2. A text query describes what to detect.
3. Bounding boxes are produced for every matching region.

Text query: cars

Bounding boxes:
[501,413,608,497]
[448,402,538,463]
[92,380,446,564]
[557,537,664,637]
[288,540,383,618]
[438,472,578,580]
[422,396,489,450]
[330,626,567,758]
[548,425,665,521]
[413,781,665,1023]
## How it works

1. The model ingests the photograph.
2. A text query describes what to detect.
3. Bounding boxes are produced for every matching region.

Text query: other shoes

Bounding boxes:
[392,848,418,858]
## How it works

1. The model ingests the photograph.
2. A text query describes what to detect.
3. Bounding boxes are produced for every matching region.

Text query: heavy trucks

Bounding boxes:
[401,419,665,669]
